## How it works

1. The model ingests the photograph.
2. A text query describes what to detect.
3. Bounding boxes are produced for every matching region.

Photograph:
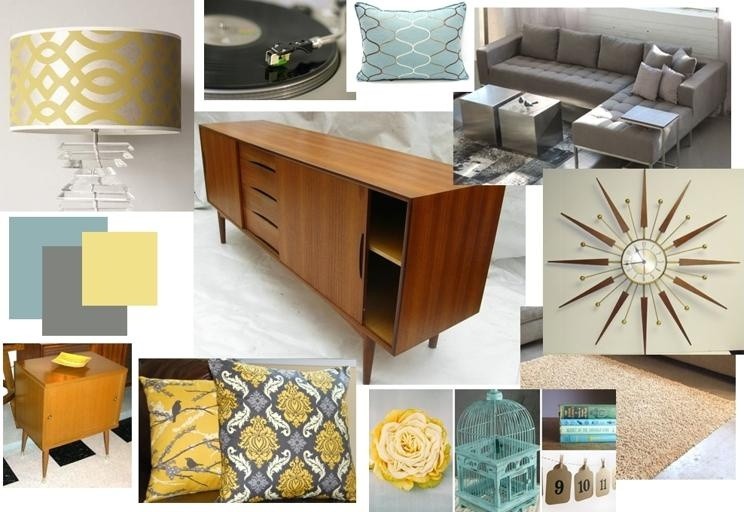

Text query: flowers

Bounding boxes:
[372,408,453,493]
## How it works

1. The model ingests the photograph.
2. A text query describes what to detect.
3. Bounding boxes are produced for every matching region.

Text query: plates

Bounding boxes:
[50,350,91,368]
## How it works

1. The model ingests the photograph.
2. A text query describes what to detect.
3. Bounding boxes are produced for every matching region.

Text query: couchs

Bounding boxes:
[479,22,727,168]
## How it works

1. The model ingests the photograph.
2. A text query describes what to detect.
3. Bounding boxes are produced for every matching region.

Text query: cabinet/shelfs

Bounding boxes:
[198,118,506,383]
[13,350,128,480]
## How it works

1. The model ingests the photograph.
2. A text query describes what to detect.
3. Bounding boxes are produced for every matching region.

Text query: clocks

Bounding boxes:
[546,169,742,353]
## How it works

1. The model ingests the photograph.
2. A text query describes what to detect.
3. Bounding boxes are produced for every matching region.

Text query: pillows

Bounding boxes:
[630,62,663,100]
[660,63,684,104]
[138,374,220,503]
[209,357,356,504]
[670,49,699,79]
[644,44,673,69]
[354,1,470,81]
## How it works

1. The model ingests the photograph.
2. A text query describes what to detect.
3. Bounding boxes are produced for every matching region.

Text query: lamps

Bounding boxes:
[10,27,182,211]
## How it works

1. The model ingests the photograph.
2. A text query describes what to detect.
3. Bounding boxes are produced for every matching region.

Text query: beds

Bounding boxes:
[458,82,561,156]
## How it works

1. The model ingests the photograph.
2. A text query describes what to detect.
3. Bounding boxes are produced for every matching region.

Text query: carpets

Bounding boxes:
[453,118,585,184]
[520,353,735,481]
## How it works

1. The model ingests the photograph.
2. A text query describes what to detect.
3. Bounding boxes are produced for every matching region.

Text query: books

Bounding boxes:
[619,103,678,131]
